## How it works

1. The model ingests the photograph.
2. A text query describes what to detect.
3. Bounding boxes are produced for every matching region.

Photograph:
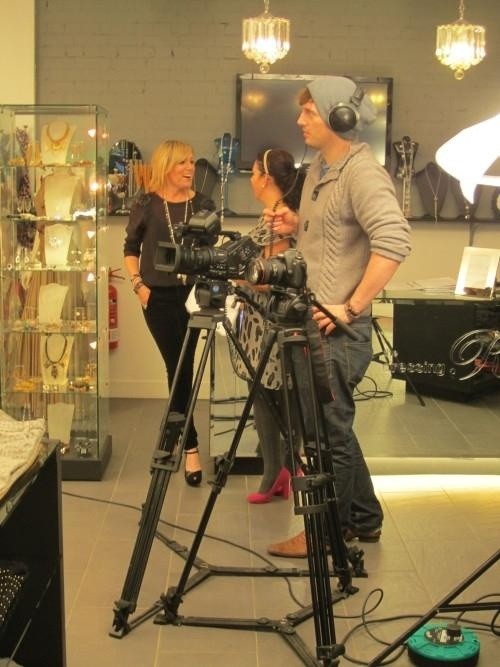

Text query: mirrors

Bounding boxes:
[455,248,500,295]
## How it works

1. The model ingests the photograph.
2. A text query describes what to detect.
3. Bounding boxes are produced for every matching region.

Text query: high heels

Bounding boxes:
[185,448,204,487]
[246,465,306,503]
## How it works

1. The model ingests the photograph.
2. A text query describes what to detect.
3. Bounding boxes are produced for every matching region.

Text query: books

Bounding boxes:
[0,411,48,501]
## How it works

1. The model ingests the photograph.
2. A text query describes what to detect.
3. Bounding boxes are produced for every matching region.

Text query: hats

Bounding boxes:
[307,75,376,141]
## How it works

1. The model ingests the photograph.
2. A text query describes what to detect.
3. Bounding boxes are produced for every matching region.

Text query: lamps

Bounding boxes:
[433,0,486,79]
[239,0,292,75]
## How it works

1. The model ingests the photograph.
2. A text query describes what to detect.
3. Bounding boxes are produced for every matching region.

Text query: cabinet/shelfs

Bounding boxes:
[0,458,66,667]
[0,104,115,483]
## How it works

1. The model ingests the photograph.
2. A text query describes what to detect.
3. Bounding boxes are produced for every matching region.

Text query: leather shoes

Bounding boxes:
[267,522,384,557]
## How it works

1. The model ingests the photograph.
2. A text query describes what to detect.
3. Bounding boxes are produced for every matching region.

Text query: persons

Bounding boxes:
[230,146,307,504]
[123,141,220,488]
[264,73,414,560]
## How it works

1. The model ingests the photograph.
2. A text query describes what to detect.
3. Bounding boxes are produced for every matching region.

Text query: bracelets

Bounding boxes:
[343,299,361,322]
[129,273,141,283]
[132,280,143,294]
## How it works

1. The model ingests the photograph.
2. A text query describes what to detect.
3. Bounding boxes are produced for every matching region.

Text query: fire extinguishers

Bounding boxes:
[108,267,125,350]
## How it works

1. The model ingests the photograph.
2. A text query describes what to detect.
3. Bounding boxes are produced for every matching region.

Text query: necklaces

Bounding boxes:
[42,333,68,378]
[425,167,442,222]
[193,163,209,193]
[111,146,137,174]
[187,198,195,217]
[44,223,70,249]
[462,198,471,221]
[162,192,190,284]
[220,136,233,221]
[46,119,71,152]
[400,138,415,218]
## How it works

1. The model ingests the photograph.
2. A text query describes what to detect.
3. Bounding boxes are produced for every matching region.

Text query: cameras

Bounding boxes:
[247,248,308,291]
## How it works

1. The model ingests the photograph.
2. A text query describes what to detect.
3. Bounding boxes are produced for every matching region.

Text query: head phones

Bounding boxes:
[328,75,365,133]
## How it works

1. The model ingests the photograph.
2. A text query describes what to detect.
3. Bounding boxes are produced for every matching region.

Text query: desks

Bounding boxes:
[393,301,499,403]
